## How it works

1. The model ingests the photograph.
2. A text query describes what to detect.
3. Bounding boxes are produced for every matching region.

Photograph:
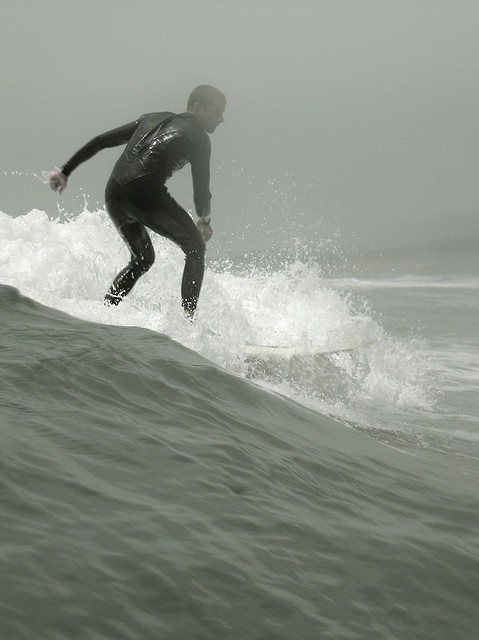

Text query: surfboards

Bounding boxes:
[216,314,381,356]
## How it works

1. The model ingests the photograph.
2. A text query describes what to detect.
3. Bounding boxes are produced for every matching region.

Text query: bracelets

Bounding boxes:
[197,217,211,226]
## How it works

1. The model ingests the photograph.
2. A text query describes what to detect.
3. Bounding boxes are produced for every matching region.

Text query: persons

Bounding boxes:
[49,86,227,319]
